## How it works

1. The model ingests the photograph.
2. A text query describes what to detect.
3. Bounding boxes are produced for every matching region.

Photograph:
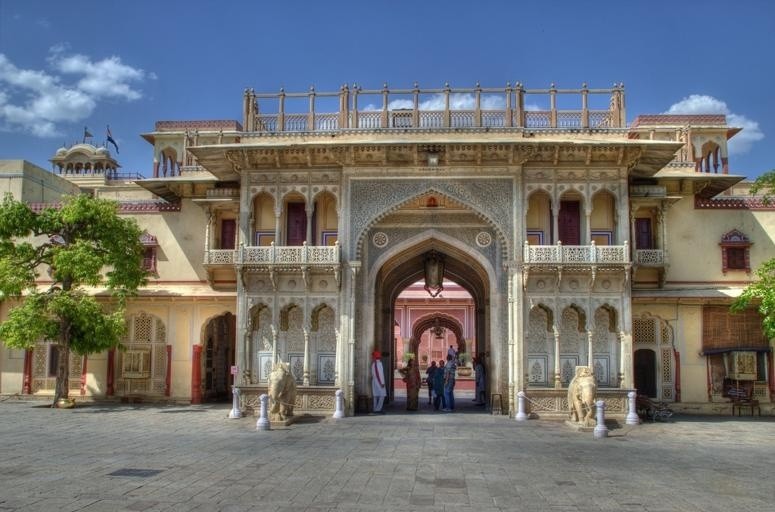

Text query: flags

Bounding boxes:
[107,126,119,154]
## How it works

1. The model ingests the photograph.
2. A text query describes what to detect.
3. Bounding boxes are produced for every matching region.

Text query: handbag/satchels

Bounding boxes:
[402,377,409,383]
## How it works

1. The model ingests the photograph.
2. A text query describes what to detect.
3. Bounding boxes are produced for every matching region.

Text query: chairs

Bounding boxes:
[637,394,674,423]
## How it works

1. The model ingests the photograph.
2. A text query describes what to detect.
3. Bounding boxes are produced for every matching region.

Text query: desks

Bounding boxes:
[732,400,760,418]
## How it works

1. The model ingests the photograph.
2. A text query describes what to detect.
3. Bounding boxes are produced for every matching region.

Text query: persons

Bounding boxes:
[398,358,422,412]
[370,350,389,413]
[425,344,464,413]
[472,355,486,403]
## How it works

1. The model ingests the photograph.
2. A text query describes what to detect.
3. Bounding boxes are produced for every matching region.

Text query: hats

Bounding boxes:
[371,351,381,359]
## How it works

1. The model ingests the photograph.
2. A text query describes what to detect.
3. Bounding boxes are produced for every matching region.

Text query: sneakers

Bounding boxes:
[373,410,386,415]
[406,407,418,411]
[426,402,457,412]
